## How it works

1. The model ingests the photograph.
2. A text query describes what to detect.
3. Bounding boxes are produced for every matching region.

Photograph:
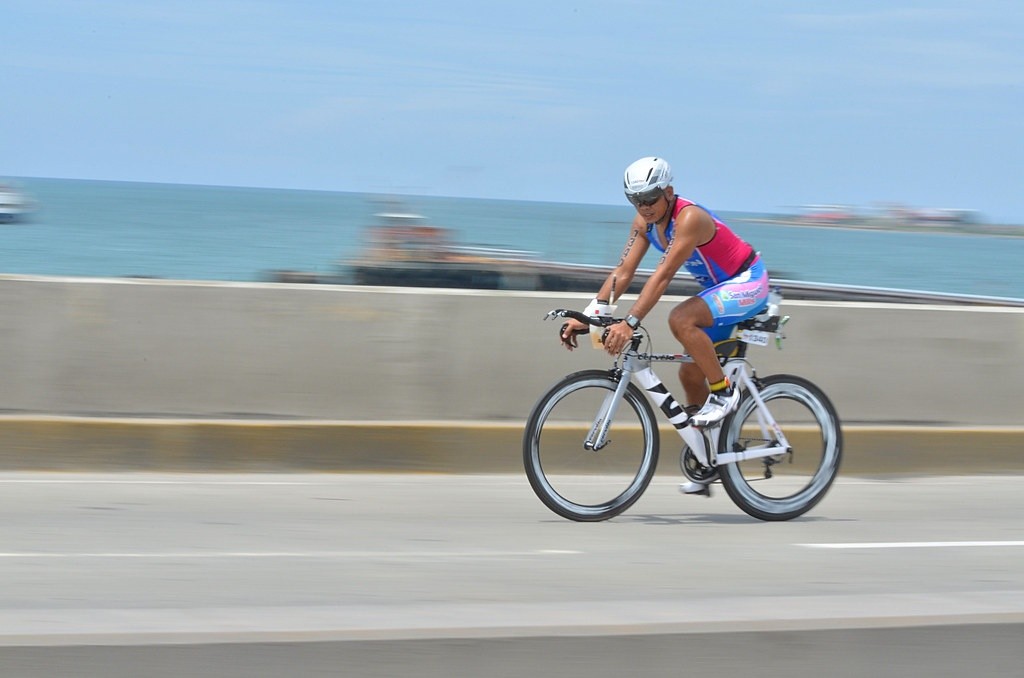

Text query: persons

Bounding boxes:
[559,156,770,494]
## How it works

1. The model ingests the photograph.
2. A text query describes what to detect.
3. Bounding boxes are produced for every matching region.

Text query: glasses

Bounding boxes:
[625,192,663,208]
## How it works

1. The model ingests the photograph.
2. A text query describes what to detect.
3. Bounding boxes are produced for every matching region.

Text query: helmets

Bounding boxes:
[623,156,674,196]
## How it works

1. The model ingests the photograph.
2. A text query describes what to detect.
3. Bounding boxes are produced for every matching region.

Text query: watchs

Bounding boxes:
[624,314,640,330]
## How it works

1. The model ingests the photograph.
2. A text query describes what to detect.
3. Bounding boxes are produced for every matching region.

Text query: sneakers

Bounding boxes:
[677,471,707,493]
[688,384,741,427]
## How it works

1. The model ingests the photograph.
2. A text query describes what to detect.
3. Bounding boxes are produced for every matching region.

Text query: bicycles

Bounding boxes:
[522,302,845,521]
